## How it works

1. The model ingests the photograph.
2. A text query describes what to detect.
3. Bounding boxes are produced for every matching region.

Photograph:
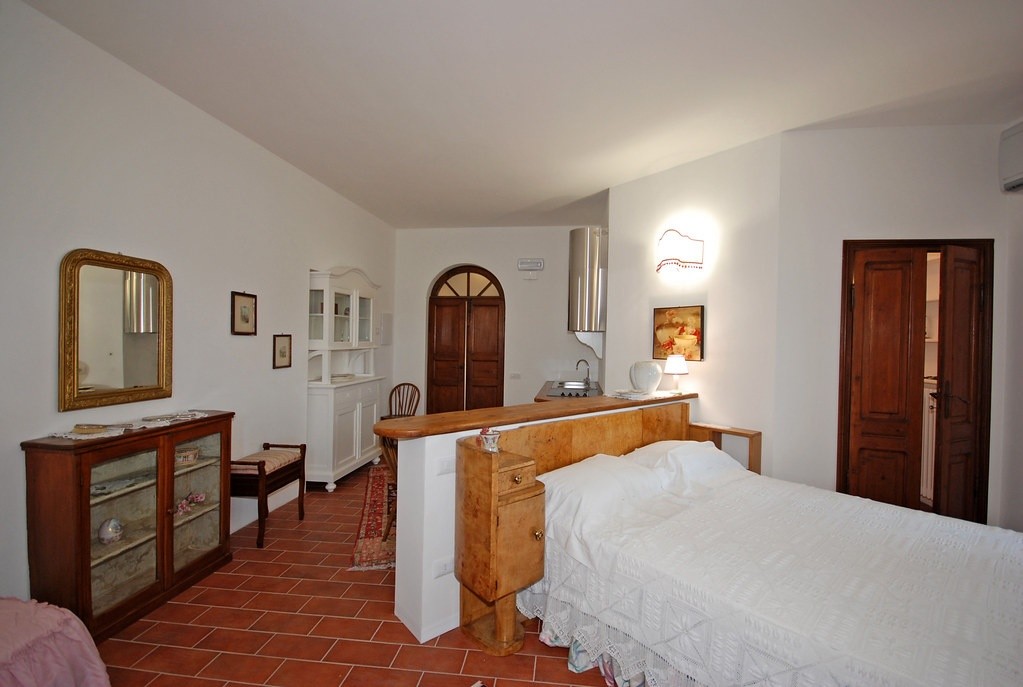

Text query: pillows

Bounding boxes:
[535,440,757,579]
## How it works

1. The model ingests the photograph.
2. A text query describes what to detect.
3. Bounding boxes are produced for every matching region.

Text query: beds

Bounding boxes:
[455,400,1022,687]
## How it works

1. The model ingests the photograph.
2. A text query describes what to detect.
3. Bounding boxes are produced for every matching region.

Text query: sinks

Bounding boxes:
[563,385,586,388]
[564,381,588,386]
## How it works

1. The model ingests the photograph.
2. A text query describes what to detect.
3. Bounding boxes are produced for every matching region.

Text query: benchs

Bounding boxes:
[230,443,307,548]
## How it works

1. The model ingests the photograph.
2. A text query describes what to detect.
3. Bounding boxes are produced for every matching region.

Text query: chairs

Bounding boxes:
[379,436,398,541]
[379,383,420,445]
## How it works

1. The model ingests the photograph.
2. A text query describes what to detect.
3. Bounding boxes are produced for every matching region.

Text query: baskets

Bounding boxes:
[174,447,200,466]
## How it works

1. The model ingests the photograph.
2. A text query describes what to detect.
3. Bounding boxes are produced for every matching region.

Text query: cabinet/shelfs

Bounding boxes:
[309,266,385,493]
[20,410,236,646]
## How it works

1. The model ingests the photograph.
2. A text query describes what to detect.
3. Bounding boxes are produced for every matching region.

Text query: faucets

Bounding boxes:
[576,359,591,386]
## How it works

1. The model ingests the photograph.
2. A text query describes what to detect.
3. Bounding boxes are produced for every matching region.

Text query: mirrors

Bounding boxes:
[57,248,173,412]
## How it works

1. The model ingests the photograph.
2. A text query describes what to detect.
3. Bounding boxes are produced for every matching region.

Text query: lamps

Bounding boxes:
[656,230,704,273]
[664,354,689,395]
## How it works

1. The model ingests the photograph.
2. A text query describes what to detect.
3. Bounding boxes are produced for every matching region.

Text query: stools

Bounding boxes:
[0,596,112,687]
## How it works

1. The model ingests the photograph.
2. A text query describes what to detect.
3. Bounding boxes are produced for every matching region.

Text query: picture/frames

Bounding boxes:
[273,334,292,369]
[652,305,704,361]
[231,291,257,335]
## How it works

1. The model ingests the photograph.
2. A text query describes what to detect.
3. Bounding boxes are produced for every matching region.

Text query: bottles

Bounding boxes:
[480,431,500,458]
[629,360,662,393]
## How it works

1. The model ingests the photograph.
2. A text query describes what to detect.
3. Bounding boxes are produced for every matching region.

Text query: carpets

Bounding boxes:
[346,465,397,572]
